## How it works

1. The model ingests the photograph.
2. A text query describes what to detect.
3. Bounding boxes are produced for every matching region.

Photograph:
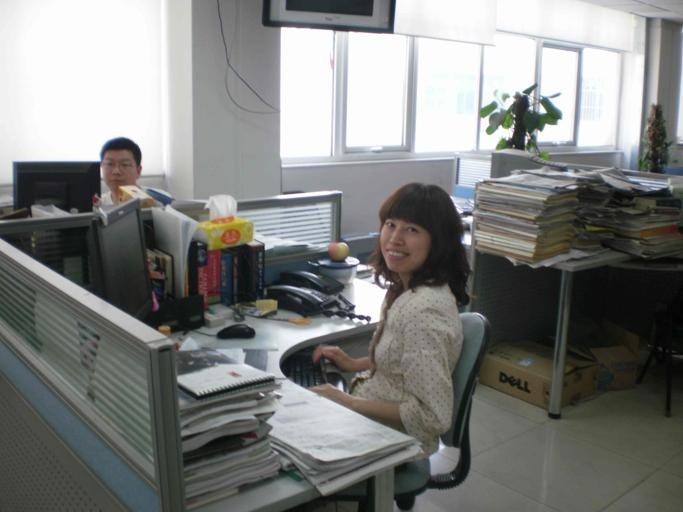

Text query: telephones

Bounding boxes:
[266,269,345,316]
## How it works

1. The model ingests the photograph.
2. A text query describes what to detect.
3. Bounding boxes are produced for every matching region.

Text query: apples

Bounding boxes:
[328,242,349,262]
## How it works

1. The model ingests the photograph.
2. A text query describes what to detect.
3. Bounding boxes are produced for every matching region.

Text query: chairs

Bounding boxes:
[633,281,683,417]
[319,309,490,512]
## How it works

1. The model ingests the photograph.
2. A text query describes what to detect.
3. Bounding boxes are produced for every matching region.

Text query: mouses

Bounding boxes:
[217,324,255,338]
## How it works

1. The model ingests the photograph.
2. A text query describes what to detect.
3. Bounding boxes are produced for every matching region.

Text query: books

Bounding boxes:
[184,240,265,311]
[472,167,683,265]
[174,347,281,511]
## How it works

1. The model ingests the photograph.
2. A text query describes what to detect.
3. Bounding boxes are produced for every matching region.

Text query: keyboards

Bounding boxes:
[281,354,326,389]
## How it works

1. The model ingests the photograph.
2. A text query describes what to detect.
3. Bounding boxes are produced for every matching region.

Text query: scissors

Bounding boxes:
[274,318,311,324]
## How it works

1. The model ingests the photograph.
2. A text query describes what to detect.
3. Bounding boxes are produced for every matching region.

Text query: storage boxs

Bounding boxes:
[481,334,597,413]
[552,313,640,395]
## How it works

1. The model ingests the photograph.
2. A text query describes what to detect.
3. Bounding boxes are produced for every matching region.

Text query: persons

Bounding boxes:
[288,183,473,512]
[100,137,170,205]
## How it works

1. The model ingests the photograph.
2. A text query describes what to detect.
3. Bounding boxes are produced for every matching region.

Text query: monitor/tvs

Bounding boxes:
[13,161,101,212]
[92,197,153,321]
[491,149,568,178]
[262,0,396,33]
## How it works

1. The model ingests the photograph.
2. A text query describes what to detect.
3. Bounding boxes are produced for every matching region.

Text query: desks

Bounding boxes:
[478,230,633,418]
[34,266,426,512]
